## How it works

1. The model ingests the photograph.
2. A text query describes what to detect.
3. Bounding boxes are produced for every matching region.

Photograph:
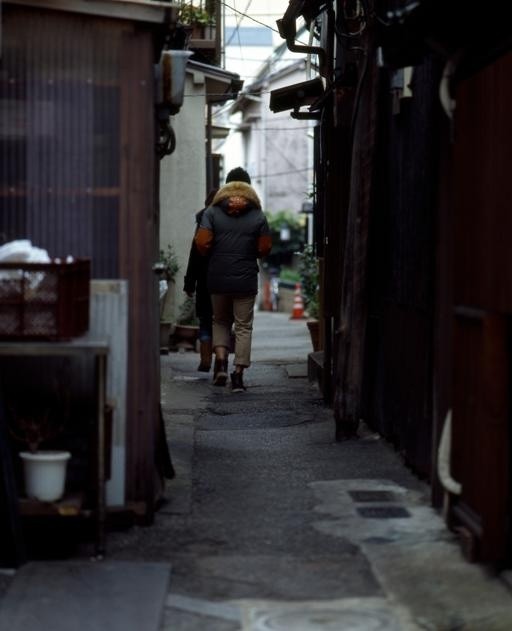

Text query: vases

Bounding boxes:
[19,450,72,503]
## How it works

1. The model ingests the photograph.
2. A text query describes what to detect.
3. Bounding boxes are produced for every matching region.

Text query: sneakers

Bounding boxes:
[212,357,228,386]
[231,371,245,392]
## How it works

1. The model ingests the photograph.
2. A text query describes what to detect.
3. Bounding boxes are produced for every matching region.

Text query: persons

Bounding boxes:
[179,187,220,374]
[194,166,272,396]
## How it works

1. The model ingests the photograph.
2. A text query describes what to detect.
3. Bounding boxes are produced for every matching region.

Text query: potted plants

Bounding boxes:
[159,243,180,350]
[307,285,320,351]
[182,4,208,39]
[174,296,200,349]
[204,15,217,40]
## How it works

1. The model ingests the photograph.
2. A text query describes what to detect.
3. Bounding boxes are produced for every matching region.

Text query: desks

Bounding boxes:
[0,336,109,561]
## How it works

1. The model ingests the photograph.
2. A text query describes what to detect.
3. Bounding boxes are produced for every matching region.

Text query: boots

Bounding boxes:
[197,340,212,372]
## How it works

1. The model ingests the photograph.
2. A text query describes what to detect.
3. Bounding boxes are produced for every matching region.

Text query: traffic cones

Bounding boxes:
[285,282,309,322]
[258,280,274,311]
